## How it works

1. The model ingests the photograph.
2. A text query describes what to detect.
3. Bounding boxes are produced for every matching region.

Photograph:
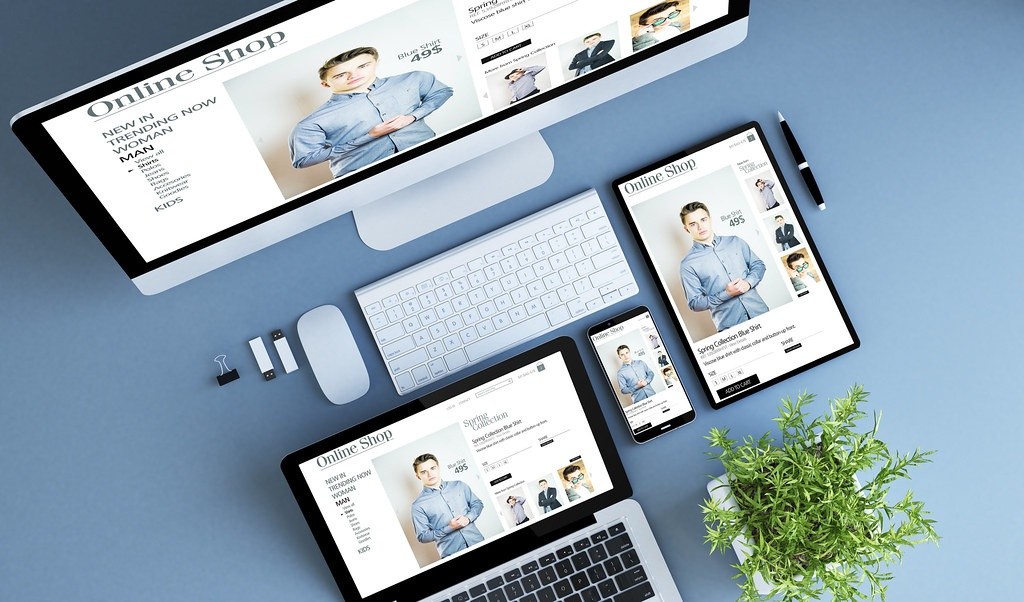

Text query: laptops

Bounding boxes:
[279,336,684,602]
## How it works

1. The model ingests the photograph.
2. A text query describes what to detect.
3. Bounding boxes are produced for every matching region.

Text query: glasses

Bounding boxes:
[667,372,673,377]
[641,10,682,26]
[792,261,809,272]
[567,473,584,484]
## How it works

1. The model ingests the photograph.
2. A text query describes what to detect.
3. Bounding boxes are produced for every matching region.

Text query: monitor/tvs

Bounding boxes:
[11,0,750,296]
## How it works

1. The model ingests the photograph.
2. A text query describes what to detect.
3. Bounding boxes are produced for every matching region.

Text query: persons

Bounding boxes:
[563,464,593,502]
[755,177,781,211]
[506,67,546,105]
[648,328,680,389]
[537,478,561,513]
[614,345,658,404]
[567,33,617,78]
[770,211,802,254]
[507,495,530,527]
[411,454,485,557]
[288,46,455,182]
[677,200,771,333]
[788,251,821,297]
[632,2,687,57]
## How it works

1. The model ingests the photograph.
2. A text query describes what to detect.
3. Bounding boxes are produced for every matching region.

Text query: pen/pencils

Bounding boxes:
[777,110,826,211]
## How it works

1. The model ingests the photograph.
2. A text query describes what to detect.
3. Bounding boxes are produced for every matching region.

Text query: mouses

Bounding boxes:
[297,304,370,405]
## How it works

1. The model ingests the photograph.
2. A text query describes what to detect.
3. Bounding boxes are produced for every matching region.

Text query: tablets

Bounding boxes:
[612,120,861,410]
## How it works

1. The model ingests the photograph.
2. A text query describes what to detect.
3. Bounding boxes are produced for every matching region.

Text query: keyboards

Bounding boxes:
[354,188,639,396]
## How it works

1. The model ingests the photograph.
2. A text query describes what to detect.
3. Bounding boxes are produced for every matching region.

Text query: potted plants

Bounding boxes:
[697,382,943,602]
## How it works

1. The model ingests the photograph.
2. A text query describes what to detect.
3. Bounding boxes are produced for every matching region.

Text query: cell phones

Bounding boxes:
[586,305,697,445]
